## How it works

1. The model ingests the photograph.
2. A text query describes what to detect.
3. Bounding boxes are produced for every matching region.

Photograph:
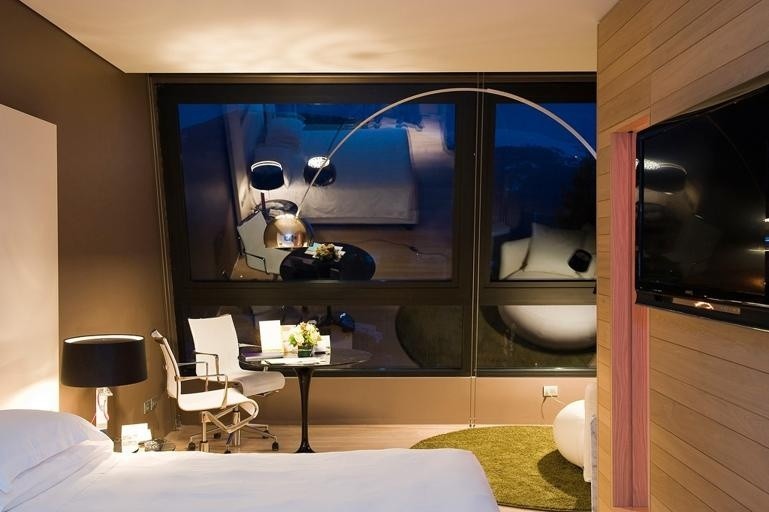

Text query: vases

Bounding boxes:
[318,255,334,268]
[296,345,314,359]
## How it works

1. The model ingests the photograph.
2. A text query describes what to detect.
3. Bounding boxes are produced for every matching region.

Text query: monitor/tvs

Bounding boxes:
[634,69,769,332]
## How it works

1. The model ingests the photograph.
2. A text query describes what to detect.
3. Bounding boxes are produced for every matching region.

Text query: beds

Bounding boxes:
[250,127,418,231]
[8,447,480,512]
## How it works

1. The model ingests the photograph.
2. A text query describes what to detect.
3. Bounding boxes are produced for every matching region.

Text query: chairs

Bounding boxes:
[552,377,598,483]
[496,236,598,351]
[149,313,286,453]
[236,209,296,281]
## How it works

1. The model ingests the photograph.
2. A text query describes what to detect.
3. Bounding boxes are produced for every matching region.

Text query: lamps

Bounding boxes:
[263,86,597,250]
[303,121,345,187]
[61,333,148,441]
[248,160,286,226]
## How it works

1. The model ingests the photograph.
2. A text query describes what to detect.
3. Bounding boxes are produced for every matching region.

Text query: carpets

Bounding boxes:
[393,299,597,370]
[411,425,591,512]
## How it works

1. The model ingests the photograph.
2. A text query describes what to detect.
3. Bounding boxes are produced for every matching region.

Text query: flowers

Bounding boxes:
[314,243,340,262]
[287,320,322,345]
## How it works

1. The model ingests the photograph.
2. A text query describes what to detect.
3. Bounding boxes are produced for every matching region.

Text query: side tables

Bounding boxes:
[254,199,298,219]
[113,433,176,454]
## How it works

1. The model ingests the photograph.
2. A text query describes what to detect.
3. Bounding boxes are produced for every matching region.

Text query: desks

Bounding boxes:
[238,348,374,453]
[279,241,376,327]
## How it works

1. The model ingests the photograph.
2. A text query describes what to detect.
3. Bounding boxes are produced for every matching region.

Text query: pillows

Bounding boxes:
[252,115,306,188]
[0,402,114,512]
[523,219,598,280]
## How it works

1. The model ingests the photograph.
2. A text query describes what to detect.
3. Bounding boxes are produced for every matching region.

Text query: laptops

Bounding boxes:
[241,352,284,361]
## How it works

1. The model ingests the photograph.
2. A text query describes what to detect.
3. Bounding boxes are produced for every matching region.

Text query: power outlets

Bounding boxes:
[144,399,151,416]
[544,384,561,397]
